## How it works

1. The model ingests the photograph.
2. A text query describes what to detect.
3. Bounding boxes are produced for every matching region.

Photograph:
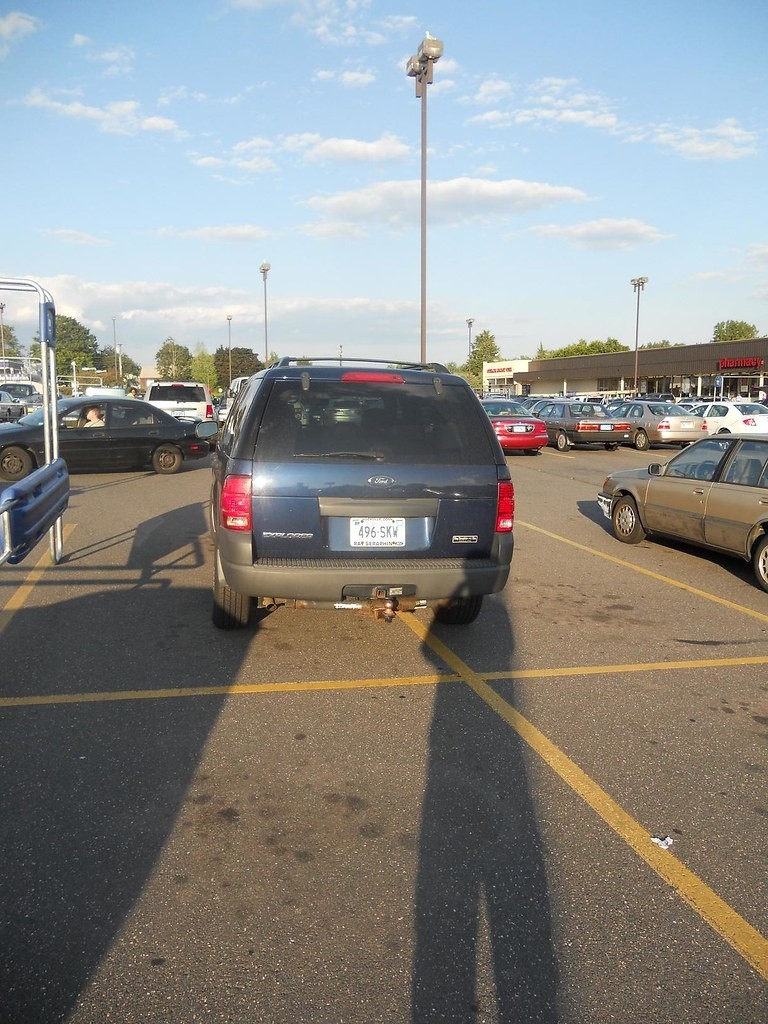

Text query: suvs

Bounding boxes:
[142,381,217,424]
[226,374,250,419]
[194,356,515,628]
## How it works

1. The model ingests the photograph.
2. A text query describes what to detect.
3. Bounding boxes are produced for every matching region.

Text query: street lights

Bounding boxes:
[117,341,124,379]
[404,27,445,370]
[630,275,649,389]
[226,313,233,387]
[465,317,475,361]
[259,258,271,361]
[112,315,118,382]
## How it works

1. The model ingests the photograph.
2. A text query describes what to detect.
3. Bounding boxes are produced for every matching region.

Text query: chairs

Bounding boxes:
[733,457,764,485]
[77,407,90,427]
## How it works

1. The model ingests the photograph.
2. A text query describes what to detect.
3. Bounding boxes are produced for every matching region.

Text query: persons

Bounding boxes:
[727,390,742,402]
[84,408,105,427]
[127,387,137,398]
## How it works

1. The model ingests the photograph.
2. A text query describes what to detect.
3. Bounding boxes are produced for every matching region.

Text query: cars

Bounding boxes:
[480,389,768,457]
[0,394,211,481]
[596,432,768,592]
[0,383,65,423]
[213,394,228,427]
